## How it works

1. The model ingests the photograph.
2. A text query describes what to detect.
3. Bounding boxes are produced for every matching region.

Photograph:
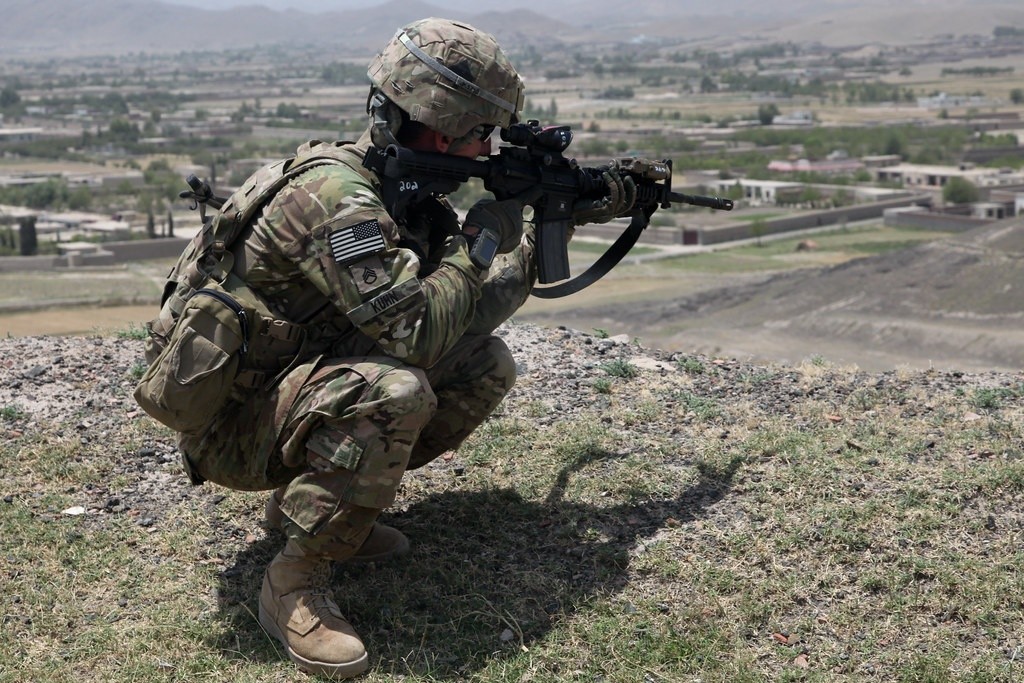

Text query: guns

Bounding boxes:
[380,116,737,286]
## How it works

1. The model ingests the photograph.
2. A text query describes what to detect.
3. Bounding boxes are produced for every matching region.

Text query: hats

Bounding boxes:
[367,16,526,138]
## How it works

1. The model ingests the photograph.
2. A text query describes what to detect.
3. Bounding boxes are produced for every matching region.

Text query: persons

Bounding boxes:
[133,19,672,680]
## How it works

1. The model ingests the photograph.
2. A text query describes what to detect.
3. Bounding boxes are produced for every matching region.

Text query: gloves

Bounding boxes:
[462,187,543,254]
[568,167,637,228]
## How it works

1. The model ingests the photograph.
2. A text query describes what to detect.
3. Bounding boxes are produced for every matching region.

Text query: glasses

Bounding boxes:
[468,123,496,144]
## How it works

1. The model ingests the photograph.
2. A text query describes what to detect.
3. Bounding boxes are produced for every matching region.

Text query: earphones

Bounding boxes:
[441,135,450,144]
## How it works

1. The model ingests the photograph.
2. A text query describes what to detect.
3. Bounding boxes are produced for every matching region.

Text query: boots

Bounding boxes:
[266,492,409,563]
[258,539,368,679]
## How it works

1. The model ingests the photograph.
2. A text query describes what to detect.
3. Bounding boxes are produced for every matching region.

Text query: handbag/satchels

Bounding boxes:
[133,288,252,437]
